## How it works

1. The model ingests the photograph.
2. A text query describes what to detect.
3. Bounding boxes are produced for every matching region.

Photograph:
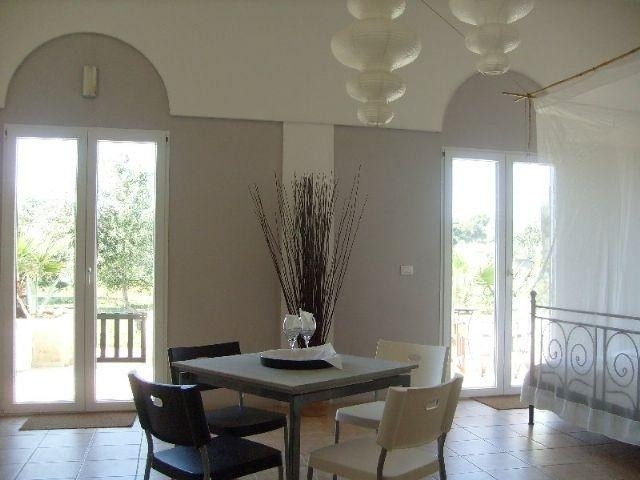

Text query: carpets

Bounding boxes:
[18,411,137,431]
[470,395,530,410]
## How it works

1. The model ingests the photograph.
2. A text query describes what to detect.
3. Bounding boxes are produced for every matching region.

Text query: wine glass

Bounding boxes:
[282,316,317,352]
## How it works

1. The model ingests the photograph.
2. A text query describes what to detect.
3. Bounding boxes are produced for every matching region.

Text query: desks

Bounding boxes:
[171,349,419,480]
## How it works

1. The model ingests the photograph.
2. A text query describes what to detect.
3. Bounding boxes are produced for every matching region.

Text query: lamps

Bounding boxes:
[330,1,535,127]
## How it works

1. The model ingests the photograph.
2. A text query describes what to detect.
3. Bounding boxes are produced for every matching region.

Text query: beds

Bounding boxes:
[523,291,640,445]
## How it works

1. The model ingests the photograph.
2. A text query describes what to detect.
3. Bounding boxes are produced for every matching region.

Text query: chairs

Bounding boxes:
[129,369,283,479]
[330,339,452,480]
[166,340,290,453]
[307,371,464,479]
